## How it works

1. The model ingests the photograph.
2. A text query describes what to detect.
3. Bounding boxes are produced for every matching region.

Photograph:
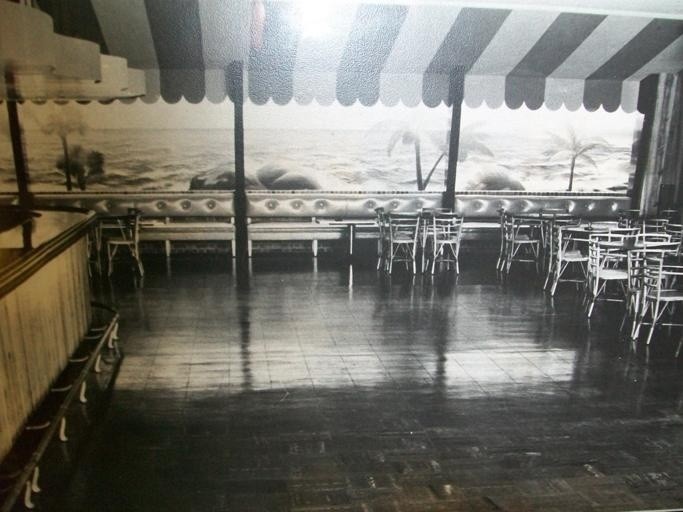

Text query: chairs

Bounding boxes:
[376,209,467,280]
[496,207,681,357]
[87,210,147,281]
